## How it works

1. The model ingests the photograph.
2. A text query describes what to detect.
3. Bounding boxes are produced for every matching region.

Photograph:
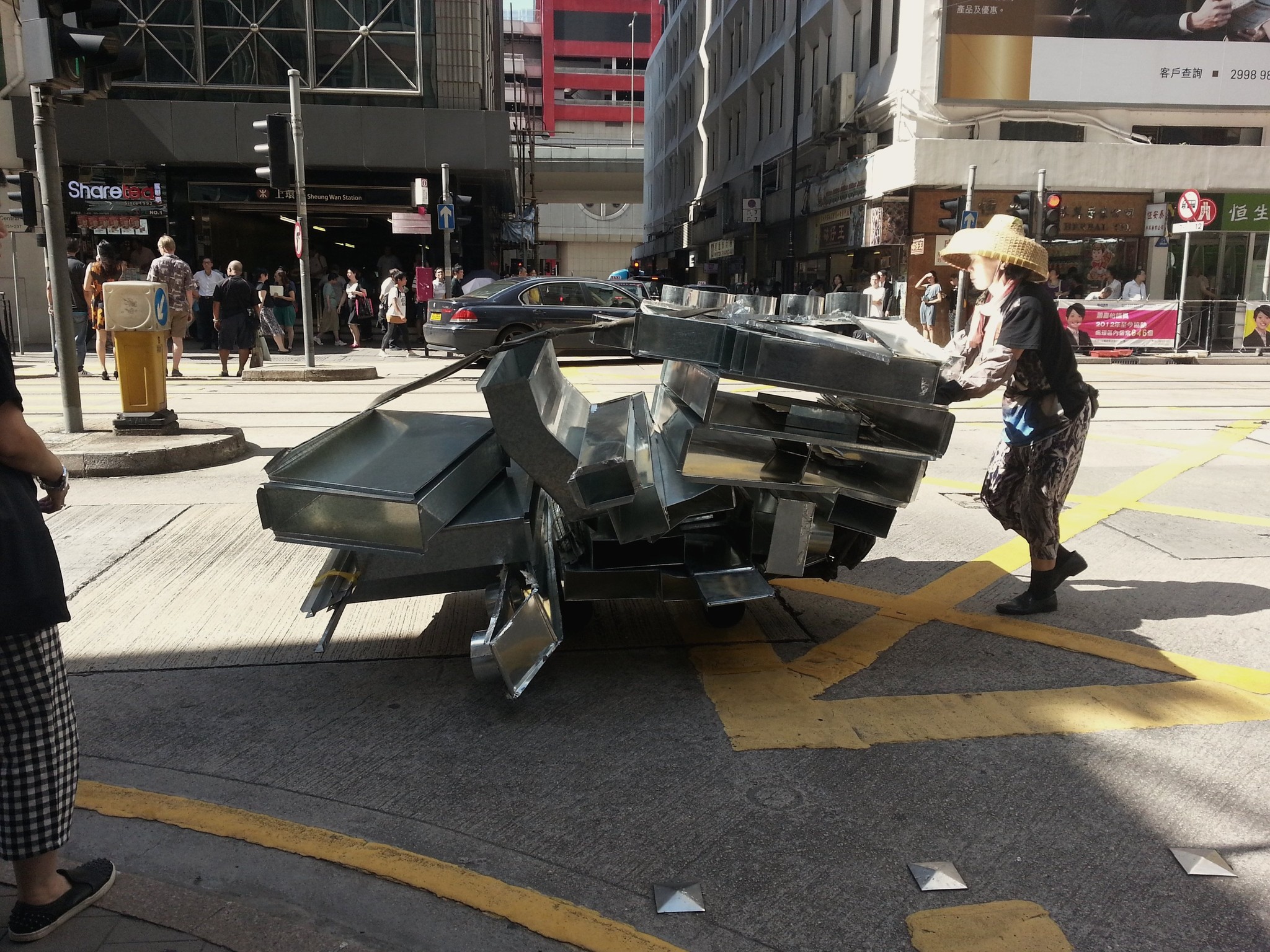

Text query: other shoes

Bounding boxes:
[165,368,168,377]
[236,371,242,377]
[186,333,193,339]
[995,591,1059,615]
[78,369,92,377]
[200,342,212,350]
[54,371,60,377]
[7,857,115,940]
[171,370,182,377]
[222,370,228,376]
[1053,551,1088,590]
[260,334,425,357]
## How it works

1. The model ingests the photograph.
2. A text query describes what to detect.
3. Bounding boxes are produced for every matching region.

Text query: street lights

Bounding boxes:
[627,10,638,147]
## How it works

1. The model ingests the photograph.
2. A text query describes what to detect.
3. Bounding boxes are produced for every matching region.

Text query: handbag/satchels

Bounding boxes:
[356,292,374,319]
[378,303,388,319]
[255,330,272,362]
[249,330,263,369]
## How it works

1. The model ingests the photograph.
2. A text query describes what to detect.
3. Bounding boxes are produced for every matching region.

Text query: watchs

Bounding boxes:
[36,464,69,491]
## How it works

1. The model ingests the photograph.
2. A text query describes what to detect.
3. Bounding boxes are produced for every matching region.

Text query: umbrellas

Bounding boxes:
[459,268,501,295]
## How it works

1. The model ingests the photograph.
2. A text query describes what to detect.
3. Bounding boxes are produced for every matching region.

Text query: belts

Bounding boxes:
[200,296,213,299]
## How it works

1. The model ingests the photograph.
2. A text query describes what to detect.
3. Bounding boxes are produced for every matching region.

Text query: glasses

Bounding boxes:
[157,245,166,252]
[203,261,212,265]
[273,275,279,278]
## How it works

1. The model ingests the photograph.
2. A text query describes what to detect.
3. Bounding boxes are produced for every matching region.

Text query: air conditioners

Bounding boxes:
[829,71,856,131]
[856,133,877,155]
[811,85,829,141]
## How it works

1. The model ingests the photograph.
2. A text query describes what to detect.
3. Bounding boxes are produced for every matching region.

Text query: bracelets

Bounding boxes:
[189,311,192,313]
[47,305,53,307]
[189,307,192,309]
[89,308,91,309]
[213,319,220,322]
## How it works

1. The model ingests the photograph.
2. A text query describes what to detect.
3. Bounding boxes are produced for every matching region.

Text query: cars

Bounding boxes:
[604,274,753,301]
[422,275,658,369]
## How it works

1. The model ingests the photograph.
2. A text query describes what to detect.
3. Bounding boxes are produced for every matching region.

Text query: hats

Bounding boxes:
[939,214,1049,283]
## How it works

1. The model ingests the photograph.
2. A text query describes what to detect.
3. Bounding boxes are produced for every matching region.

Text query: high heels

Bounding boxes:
[114,371,118,380]
[102,371,109,380]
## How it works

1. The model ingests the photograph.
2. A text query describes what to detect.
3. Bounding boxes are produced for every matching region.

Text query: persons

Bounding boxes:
[797,274,847,335]
[1164,265,1221,346]
[863,270,893,343]
[933,213,1091,615]
[1243,305,1270,353]
[1066,0,1270,42]
[915,271,941,343]
[1065,303,1096,354]
[516,266,539,306]
[47,234,296,381]
[0,321,117,942]
[746,278,781,315]
[1043,266,1148,300]
[543,288,619,307]
[309,244,464,357]
[941,274,966,340]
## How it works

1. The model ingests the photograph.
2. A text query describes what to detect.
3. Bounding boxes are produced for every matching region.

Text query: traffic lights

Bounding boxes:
[1011,191,1036,239]
[19,0,110,95]
[253,113,291,191]
[938,195,967,236]
[1042,190,1062,244]
[4,170,38,226]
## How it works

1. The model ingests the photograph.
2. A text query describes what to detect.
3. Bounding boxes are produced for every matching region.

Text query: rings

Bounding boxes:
[61,503,66,508]
